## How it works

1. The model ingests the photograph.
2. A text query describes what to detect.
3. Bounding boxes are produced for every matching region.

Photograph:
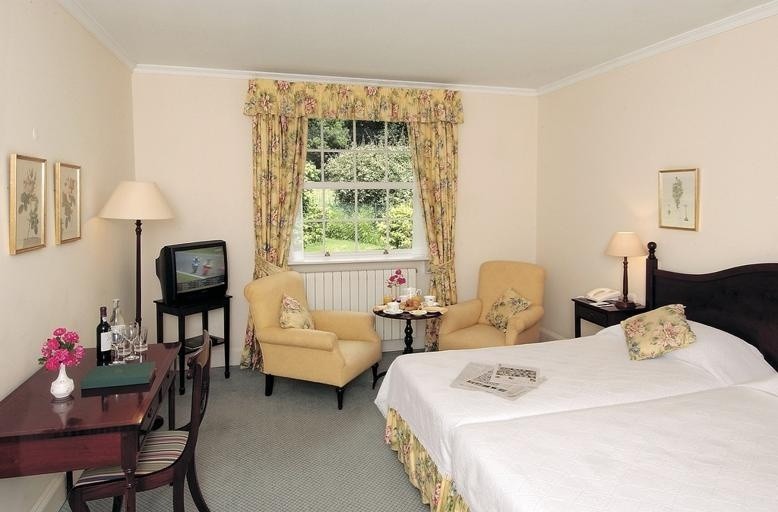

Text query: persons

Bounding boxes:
[203,258,211,274]
[192,256,200,273]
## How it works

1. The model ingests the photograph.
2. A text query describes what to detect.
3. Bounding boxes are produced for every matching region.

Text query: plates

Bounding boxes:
[383,295,439,317]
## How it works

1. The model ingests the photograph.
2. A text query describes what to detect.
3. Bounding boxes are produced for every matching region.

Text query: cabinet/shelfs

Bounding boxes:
[153,295,233,395]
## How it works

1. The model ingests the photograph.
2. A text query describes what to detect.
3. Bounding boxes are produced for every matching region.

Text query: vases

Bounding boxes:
[392,287,399,299]
[50,362,75,399]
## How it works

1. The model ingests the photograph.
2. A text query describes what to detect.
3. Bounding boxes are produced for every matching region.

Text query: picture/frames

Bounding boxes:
[55,162,82,244]
[10,153,48,255]
[657,168,700,232]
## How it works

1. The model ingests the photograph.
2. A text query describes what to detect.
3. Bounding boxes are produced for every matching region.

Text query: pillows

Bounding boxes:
[486,288,532,334]
[278,292,315,330]
[620,303,697,361]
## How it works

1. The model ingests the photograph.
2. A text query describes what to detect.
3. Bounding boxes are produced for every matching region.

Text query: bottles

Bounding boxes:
[96,307,111,366]
[110,298,124,327]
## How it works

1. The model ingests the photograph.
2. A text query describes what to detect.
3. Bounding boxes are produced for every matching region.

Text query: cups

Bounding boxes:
[116,325,131,356]
[134,327,149,352]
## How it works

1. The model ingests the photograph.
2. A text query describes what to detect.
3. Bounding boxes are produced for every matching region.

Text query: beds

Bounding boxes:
[374,240,778,512]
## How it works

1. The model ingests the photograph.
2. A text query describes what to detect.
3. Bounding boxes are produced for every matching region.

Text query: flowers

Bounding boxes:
[61,177,76,228]
[383,269,407,287]
[37,327,86,370]
[19,168,38,240]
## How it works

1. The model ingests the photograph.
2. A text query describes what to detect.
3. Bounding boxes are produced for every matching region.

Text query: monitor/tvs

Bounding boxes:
[154,241,231,302]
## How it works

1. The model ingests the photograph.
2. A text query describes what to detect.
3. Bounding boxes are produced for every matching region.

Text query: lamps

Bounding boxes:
[99,180,175,339]
[604,231,649,309]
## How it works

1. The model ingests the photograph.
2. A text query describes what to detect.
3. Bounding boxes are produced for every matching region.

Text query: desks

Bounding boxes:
[0,342,182,511]
[372,304,442,354]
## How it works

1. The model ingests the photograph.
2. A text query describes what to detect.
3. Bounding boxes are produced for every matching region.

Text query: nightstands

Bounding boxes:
[571,296,645,338]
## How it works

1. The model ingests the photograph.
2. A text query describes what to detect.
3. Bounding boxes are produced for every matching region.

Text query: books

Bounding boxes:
[80,360,155,390]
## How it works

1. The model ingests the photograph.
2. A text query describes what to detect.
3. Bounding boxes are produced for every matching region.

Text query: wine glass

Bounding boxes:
[108,327,124,366]
[123,321,139,360]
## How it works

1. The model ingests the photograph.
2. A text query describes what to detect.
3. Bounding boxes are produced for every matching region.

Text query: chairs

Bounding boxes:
[439,260,545,350]
[244,271,382,410]
[67,330,211,512]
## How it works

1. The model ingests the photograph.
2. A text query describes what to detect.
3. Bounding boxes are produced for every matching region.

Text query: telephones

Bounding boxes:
[586,288,621,303]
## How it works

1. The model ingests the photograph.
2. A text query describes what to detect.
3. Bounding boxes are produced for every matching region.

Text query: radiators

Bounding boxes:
[302,268,418,342]
